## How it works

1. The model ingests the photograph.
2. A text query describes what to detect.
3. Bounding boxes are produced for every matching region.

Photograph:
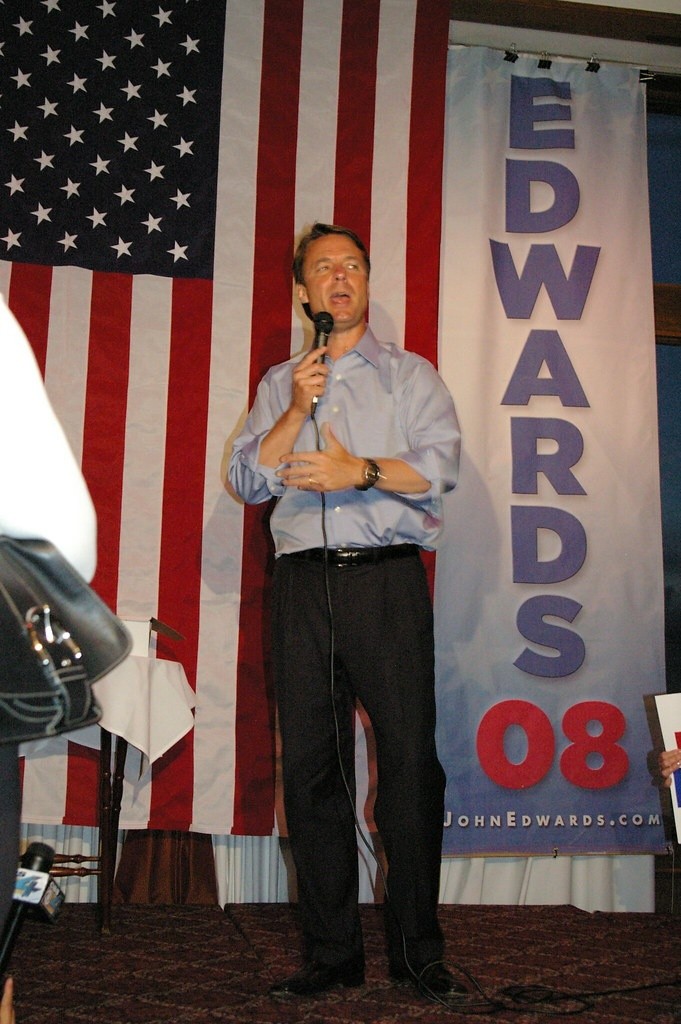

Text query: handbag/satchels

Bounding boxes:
[0,534,133,744]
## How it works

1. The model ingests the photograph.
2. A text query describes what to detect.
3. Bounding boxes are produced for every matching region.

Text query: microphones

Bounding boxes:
[0,842,66,983]
[310,311,334,416]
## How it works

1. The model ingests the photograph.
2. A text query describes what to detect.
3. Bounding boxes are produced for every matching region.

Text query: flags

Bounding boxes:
[0,0,451,836]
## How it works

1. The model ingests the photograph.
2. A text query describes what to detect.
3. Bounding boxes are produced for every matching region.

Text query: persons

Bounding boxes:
[228,222,469,1006]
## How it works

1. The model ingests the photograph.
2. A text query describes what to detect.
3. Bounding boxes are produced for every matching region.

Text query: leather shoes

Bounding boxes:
[388,955,469,998]
[268,958,365,1003]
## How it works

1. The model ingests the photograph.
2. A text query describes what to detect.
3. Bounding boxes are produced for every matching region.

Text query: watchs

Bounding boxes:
[354,457,380,491]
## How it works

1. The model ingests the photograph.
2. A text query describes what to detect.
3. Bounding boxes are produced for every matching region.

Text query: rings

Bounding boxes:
[308,474,312,485]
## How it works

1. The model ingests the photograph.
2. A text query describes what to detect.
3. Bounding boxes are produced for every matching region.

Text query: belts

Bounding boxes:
[286,544,420,567]
